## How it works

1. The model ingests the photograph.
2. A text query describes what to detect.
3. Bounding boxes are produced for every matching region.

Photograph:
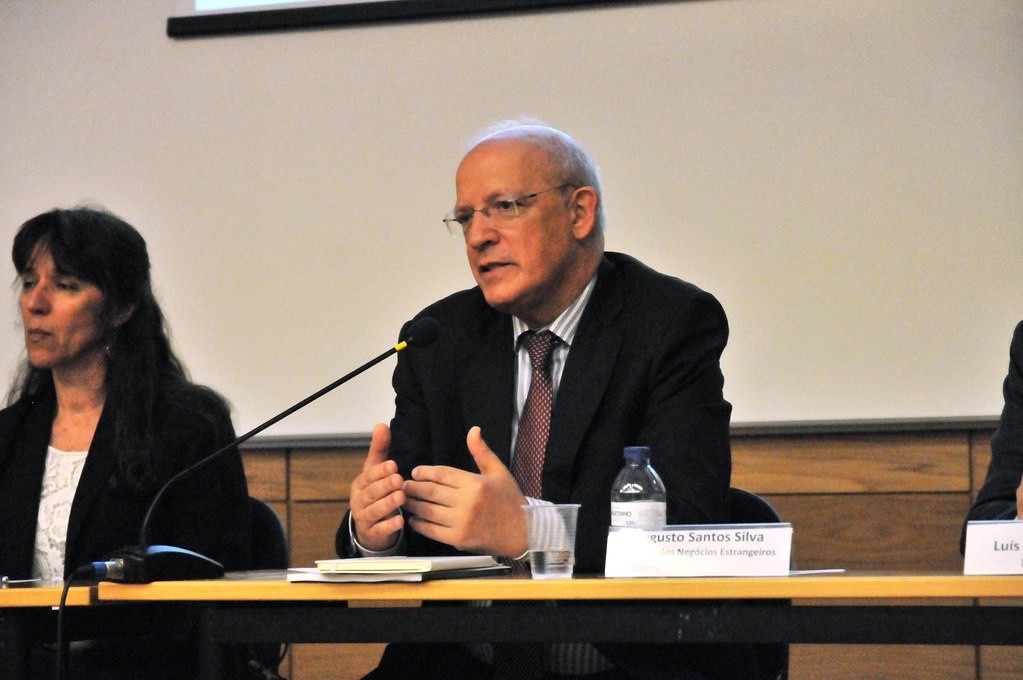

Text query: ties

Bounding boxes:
[498,331,561,576]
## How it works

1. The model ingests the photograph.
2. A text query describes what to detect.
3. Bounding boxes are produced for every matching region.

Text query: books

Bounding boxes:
[285,556,513,582]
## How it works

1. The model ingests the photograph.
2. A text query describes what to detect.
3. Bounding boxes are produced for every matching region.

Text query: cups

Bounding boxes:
[520,504,582,579]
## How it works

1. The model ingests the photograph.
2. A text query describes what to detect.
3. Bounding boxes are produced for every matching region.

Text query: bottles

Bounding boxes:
[611,446,666,526]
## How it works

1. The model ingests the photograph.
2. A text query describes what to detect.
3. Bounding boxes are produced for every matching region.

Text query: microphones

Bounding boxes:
[99,316,440,582]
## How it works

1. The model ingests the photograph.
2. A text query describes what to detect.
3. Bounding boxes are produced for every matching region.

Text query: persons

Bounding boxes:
[0,207,250,680]
[959,319,1023,558]
[333,118,733,680]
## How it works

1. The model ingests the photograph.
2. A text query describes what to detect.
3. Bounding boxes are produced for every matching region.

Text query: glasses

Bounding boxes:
[443,183,578,236]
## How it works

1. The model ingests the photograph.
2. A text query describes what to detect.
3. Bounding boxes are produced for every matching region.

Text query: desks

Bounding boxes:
[0,575,1023,680]
